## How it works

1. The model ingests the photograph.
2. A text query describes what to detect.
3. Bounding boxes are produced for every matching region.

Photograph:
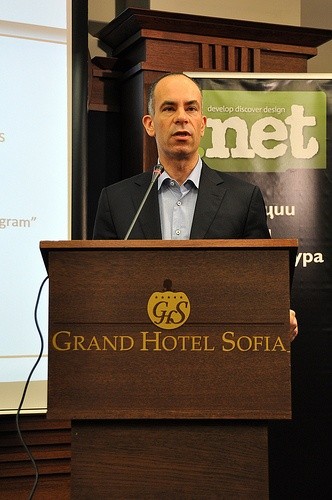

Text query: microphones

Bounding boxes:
[124,164,164,240]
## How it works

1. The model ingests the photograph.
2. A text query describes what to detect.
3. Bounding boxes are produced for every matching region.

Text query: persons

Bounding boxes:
[92,72,299,342]
